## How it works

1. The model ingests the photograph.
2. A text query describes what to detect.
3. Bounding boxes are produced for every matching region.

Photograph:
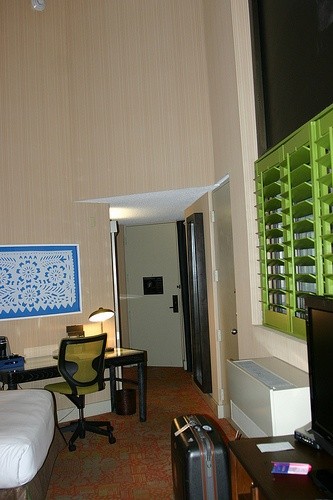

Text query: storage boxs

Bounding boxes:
[0,355,24,371]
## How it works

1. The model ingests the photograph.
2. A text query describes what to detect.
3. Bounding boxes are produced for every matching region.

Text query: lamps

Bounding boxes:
[89,307,116,352]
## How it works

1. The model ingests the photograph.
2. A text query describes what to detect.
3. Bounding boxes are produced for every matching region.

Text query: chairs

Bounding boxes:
[45,332,116,451]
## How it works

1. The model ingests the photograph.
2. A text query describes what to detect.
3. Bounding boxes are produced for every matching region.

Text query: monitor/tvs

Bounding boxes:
[304,293,333,457]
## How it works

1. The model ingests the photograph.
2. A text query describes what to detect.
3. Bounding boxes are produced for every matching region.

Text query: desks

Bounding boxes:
[226,356,312,438]
[0,346,148,422]
[227,435,333,500]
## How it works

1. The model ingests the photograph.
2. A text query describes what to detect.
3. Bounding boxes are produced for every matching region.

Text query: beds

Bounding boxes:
[0,389,57,500]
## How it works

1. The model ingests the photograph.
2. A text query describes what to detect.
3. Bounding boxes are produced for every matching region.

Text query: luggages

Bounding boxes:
[171,414,231,500]
